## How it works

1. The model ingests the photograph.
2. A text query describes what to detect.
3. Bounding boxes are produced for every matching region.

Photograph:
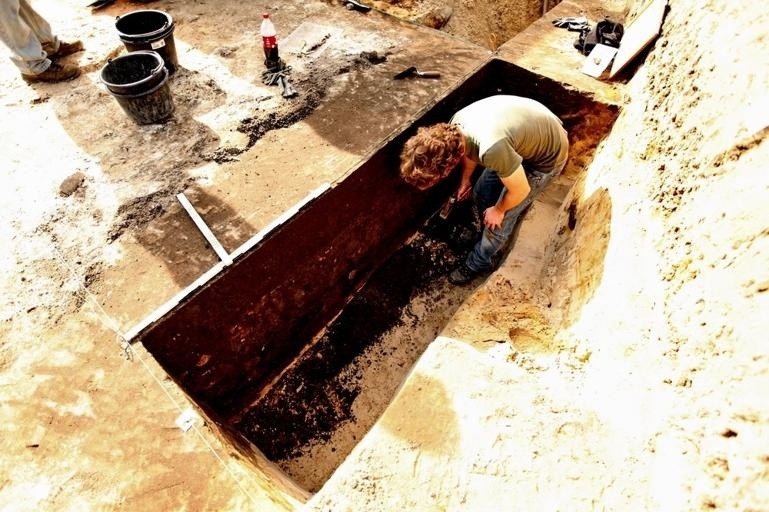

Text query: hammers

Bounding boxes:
[440,184,472,219]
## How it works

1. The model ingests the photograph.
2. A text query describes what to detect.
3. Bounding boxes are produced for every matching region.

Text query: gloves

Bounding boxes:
[551,16,590,32]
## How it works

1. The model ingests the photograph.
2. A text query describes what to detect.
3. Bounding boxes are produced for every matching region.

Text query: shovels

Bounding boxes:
[392,66,440,80]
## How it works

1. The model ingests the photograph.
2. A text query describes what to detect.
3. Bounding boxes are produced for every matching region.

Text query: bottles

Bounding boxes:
[260,12,279,69]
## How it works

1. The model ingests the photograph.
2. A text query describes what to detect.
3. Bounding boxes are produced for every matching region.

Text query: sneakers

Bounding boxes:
[48,41,83,58]
[449,265,477,284]
[21,62,81,84]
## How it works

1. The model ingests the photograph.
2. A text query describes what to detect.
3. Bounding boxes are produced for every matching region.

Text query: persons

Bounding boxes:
[397,94,570,285]
[0,0,82,84]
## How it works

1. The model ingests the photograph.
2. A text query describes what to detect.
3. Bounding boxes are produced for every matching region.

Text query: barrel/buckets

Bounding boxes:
[115,9,177,75]
[100,49,175,125]
